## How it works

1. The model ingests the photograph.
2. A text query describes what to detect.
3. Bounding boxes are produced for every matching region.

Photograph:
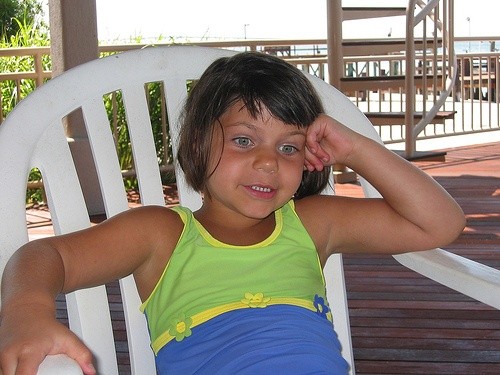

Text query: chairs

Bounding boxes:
[0,44,499,375]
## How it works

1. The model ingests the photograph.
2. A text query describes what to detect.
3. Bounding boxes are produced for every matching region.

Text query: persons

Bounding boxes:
[0,48,467,375]
